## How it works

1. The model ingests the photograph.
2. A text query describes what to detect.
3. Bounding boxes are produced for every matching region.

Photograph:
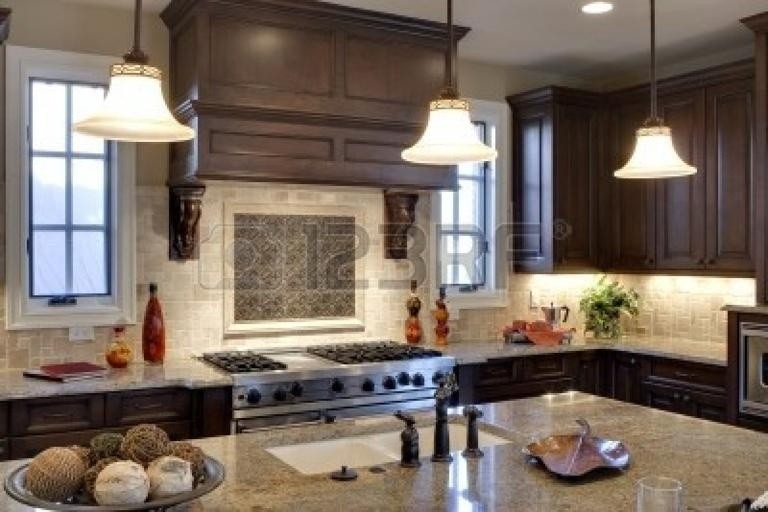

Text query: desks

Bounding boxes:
[0,387,766,512]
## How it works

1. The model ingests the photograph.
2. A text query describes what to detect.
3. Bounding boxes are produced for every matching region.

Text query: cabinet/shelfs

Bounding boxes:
[706,55,755,272]
[159,0,471,192]
[511,92,594,274]
[458,343,607,403]
[0,360,233,456]
[608,349,726,423]
[590,69,712,273]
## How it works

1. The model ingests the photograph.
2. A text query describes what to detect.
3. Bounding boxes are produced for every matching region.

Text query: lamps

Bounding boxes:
[400,0,500,166]
[75,0,195,143]
[612,0,700,180]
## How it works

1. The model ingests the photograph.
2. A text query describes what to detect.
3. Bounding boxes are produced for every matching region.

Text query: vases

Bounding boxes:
[431,285,452,352]
[101,323,134,370]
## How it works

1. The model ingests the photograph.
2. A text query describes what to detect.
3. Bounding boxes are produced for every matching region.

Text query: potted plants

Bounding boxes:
[574,274,646,343]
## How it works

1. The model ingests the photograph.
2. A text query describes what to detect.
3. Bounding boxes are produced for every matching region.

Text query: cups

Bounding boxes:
[633,475,684,512]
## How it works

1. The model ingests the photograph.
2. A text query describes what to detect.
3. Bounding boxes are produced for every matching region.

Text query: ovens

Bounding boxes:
[199,338,455,410]
[233,388,436,434]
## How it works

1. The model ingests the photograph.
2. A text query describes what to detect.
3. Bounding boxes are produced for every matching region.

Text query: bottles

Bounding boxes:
[106,326,135,368]
[433,298,450,346]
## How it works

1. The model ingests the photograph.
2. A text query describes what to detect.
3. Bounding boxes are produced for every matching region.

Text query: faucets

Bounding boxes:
[430,368,460,464]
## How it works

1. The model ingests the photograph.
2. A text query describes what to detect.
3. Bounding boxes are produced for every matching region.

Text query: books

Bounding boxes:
[42,360,110,378]
[22,369,83,382]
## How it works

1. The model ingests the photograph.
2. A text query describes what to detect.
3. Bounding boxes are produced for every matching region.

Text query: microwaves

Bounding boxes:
[739,323,767,417]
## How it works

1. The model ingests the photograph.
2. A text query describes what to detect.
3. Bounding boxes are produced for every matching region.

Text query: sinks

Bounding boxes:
[379,421,513,460]
[264,434,395,476]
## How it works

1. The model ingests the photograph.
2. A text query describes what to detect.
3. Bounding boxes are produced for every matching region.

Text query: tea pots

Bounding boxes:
[541,301,569,331]
